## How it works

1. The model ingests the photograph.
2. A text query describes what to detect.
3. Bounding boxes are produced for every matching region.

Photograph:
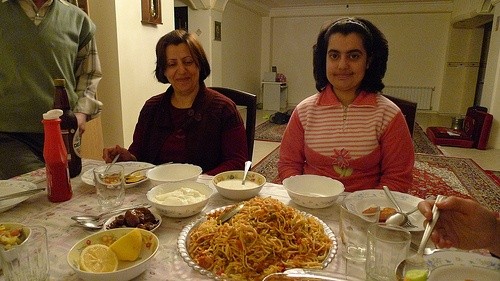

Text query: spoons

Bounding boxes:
[73,202,150,229]
[395,194,449,281]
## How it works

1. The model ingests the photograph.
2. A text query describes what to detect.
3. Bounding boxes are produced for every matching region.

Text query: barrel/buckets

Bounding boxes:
[450,114,465,130]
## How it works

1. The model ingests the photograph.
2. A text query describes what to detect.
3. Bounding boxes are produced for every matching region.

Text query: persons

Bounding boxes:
[103,29,249,179]
[418,196,500,259]
[0,0,103,179]
[277,16,415,194]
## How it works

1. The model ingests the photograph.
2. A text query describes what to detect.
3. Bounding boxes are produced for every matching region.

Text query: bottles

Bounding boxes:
[52,79,82,177]
[43,109,72,202]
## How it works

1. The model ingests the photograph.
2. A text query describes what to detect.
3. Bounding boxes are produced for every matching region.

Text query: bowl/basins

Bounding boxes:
[67,227,159,279]
[0,222,31,262]
[147,164,203,189]
[283,174,345,209]
[146,180,215,219]
[212,171,267,201]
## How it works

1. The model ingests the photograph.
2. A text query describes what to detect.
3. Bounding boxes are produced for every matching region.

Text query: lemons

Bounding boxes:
[80,244,118,273]
[108,229,142,261]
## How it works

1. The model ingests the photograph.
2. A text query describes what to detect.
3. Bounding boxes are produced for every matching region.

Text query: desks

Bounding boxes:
[261,82,288,111]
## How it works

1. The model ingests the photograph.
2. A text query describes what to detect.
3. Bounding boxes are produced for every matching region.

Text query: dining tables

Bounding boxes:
[0,157,439,281]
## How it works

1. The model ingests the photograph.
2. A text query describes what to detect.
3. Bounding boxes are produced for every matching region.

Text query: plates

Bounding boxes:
[346,189,427,231]
[177,205,338,281]
[0,180,38,213]
[82,161,156,188]
[103,212,162,235]
[424,251,500,281]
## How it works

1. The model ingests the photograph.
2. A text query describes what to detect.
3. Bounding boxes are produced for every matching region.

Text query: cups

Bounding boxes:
[364,223,413,281]
[340,196,381,262]
[92,165,127,207]
[0,225,51,281]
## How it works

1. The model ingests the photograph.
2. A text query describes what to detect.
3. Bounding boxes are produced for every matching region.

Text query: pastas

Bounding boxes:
[186,196,331,281]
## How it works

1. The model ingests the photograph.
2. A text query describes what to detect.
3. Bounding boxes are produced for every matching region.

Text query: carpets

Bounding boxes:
[251,144,500,222]
[254,109,446,157]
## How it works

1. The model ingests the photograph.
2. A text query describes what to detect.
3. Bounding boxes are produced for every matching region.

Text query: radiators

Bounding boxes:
[382,84,435,110]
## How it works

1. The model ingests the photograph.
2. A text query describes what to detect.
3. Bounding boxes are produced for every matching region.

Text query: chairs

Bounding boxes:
[381,94,418,193]
[206,86,257,171]
